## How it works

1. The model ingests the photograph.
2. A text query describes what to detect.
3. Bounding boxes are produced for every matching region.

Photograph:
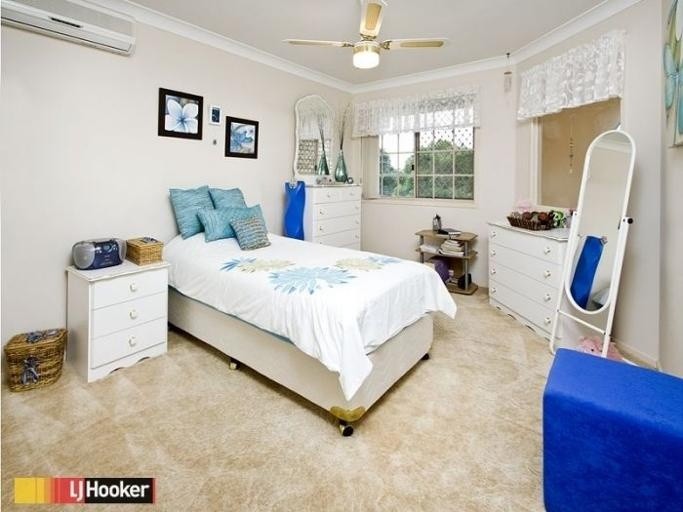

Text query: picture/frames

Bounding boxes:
[209,105,221,125]
[225,116,260,159]
[157,87,203,140]
[663,0,683,147]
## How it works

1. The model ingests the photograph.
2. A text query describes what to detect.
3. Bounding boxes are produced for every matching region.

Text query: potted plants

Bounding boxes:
[316,113,329,175]
[335,101,353,183]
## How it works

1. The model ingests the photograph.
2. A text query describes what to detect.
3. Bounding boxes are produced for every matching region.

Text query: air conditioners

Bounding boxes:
[1,0,138,56]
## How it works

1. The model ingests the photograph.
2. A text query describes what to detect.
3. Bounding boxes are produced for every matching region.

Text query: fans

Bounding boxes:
[282,0,447,51]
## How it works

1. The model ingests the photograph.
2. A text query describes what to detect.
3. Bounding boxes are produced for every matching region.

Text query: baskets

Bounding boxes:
[123,235,168,267]
[508,214,555,232]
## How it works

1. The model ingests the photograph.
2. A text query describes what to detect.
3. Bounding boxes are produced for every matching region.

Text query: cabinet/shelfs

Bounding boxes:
[413,227,478,295]
[303,184,362,250]
[486,221,568,342]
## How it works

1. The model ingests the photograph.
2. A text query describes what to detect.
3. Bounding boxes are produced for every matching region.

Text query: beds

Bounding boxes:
[165,229,458,438]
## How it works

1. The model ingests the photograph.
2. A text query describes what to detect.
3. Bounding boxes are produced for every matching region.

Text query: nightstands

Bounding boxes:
[65,258,169,384]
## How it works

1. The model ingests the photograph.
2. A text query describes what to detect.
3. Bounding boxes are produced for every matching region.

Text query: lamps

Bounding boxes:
[351,44,381,68]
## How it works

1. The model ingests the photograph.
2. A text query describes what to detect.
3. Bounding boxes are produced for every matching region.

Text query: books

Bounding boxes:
[438,238,465,255]
[440,229,461,235]
[448,268,461,286]
[419,243,439,254]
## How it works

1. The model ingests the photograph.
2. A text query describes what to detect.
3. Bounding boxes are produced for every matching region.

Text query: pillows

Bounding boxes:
[197,206,236,243]
[229,214,271,250]
[169,185,215,240]
[235,204,269,233]
[209,187,247,209]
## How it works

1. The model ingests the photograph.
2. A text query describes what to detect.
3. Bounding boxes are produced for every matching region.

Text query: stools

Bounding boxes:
[542,347,683,512]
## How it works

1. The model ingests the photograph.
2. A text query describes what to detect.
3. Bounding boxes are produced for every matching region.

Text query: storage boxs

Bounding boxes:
[124,236,164,264]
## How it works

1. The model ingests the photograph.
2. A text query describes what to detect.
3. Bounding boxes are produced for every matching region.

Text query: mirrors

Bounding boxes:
[565,130,636,314]
[535,98,621,211]
[294,94,336,185]
